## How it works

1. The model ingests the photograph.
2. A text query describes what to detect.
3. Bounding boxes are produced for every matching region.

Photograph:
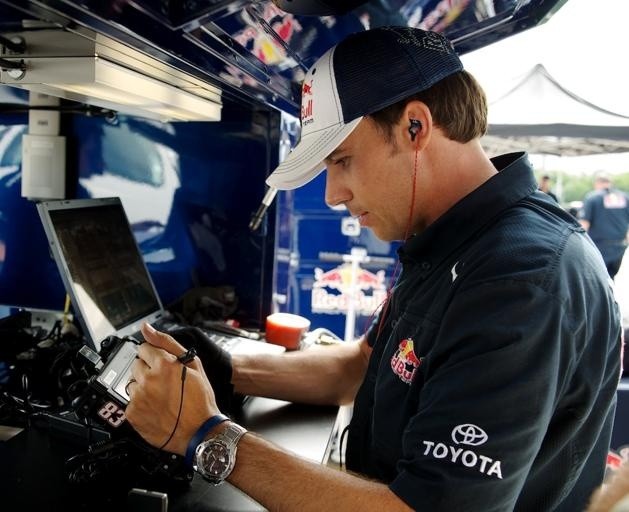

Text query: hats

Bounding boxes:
[266,27,463,190]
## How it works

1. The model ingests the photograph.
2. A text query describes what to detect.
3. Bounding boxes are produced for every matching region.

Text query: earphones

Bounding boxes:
[408,119,421,140]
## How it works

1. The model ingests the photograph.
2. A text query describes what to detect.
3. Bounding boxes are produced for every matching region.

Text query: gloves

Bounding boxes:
[165,327,232,386]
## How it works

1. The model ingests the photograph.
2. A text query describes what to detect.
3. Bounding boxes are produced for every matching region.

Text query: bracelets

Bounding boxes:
[184,413,230,465]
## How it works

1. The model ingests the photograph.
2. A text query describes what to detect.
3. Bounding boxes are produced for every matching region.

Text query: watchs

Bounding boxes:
[193,423,248,485]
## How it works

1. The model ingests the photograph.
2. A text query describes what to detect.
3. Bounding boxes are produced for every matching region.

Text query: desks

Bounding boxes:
[171,351,346,512]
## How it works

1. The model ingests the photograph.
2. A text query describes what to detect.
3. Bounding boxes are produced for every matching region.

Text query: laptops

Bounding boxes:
[34,194,288,407]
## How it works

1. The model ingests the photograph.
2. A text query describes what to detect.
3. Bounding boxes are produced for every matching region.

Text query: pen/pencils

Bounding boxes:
[178,347,198,364]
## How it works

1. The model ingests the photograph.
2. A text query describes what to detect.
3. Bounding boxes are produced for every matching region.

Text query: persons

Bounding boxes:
[575,174,629,279]
[537,176,558,204]
[125,25,622,512]
